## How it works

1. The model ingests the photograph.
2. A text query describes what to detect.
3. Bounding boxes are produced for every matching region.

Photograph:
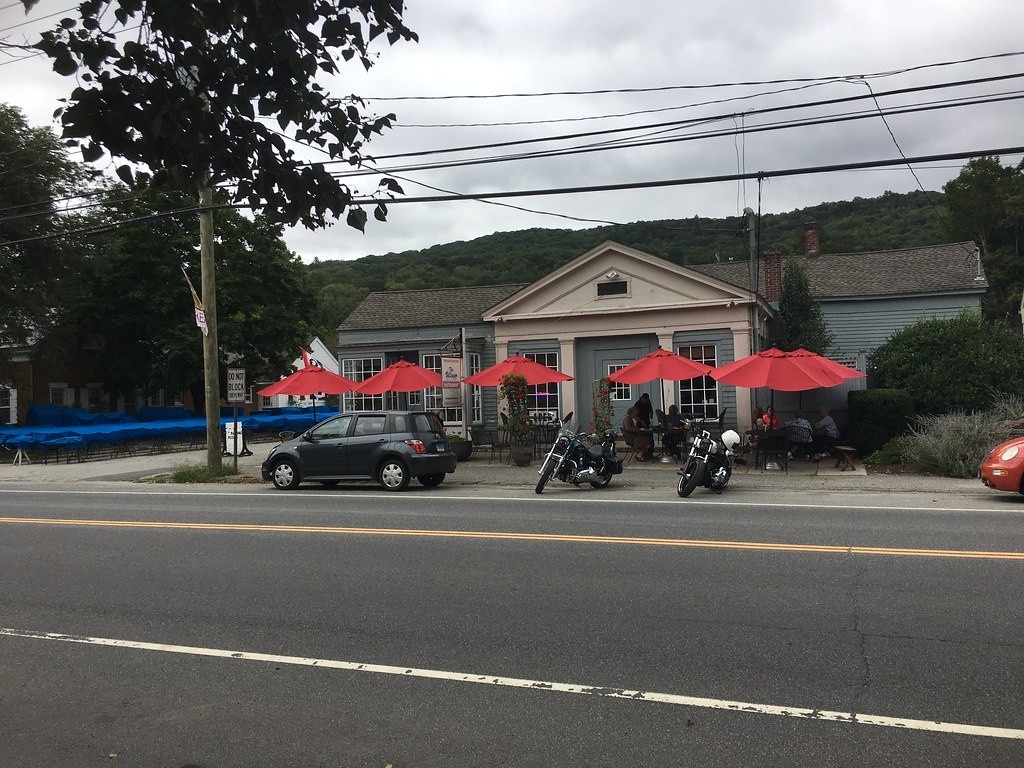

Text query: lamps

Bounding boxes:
[606,271,618,280]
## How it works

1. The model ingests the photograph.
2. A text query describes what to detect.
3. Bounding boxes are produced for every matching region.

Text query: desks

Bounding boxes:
[529,416,552,440]
[645,428,687,464]
[745,431,791,469]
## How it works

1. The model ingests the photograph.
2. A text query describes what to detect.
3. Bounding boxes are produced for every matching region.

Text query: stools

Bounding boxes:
[833,446,856,472]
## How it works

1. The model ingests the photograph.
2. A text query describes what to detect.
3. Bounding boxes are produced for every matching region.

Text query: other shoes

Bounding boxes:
[635,454,648,462]
[804,457,809,461]
[787,455,794,461]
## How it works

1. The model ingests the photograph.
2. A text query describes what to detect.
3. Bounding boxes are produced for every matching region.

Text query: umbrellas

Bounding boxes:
[605,349,715,427]
[709,349,869,432]
[351,361,442,410]
[256,365,360,427]
[463,353,576,386]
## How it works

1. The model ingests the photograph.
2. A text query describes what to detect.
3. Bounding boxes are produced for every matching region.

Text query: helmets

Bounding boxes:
[721,430,740,451]
[602,428,617,441]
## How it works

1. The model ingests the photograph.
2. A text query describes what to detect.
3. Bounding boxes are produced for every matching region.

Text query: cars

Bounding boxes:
[977,436,1024,496]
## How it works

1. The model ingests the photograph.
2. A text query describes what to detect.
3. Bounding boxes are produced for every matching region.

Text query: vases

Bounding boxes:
[511,444,533,467]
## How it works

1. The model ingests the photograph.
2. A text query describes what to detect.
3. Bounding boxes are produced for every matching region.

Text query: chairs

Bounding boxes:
[466,412,573,466]
[279,430,296,444]
[620,408,847,471]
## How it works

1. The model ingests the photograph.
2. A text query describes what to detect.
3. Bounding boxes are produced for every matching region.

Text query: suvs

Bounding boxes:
[261,409,458,492]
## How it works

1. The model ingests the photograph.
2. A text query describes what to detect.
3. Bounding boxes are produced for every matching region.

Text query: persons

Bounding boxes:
[634,393,655,459]
[785,410,812,460]
[623,407,653,461]
[749,407,781,449]
[661,405,687,460]
[815,405,839,456]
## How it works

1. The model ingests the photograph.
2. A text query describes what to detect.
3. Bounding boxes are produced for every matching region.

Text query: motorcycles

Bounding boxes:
[535,418,624,494]
[676,418,734,498]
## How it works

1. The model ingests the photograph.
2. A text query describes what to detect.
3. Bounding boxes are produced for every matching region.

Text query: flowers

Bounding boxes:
[497,373,534,444]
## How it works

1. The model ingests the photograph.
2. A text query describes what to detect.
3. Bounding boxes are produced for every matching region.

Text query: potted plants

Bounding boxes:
[447,436,472,460]
